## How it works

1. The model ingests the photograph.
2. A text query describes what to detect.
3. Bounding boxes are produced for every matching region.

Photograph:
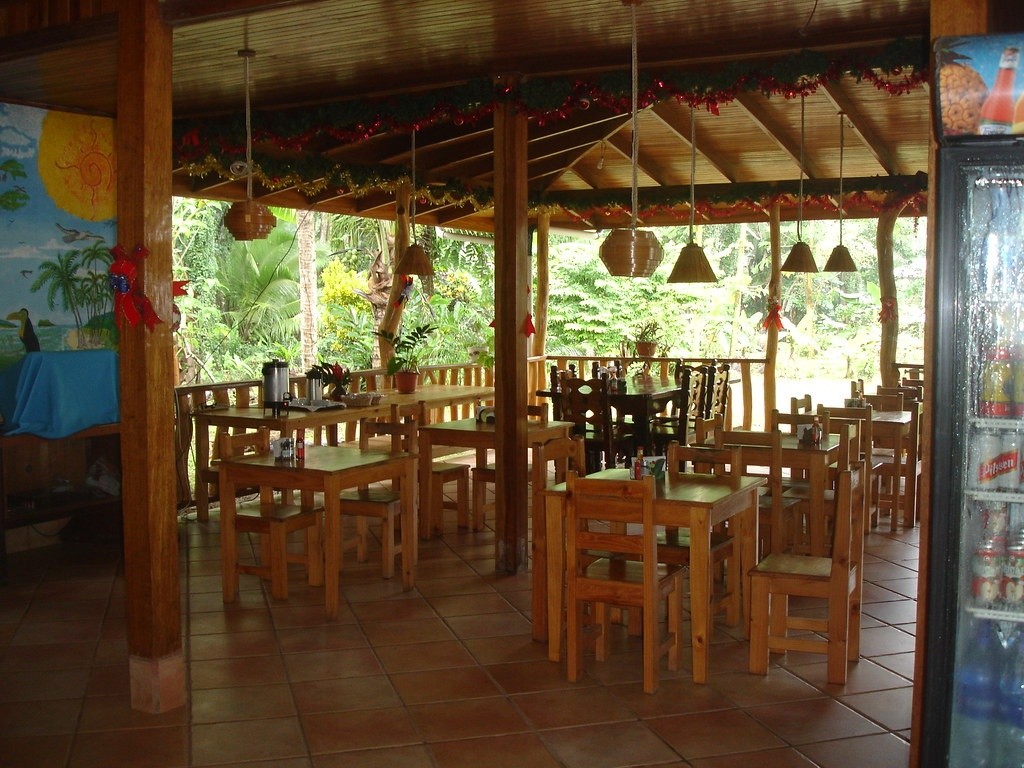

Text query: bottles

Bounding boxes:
[1013,92,1024,133]
[475,397,483,424]
[978,47,1019,134]
[274,427,305,462]
[850,390,871,408]
[600,365,625,391]
[954,618,1024,768]
[897,379,902,389]
[630,446,655,478]
[802,415,821,444]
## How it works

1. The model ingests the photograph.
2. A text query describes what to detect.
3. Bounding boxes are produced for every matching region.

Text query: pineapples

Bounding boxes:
[935,36,990,134]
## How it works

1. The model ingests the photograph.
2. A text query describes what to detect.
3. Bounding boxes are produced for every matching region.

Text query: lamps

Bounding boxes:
[822,111,859,273]
[667,102,720,283]
[598,131,665,276]
[224,48,278,242]
[780,87,819,274]
[393,127,434,277]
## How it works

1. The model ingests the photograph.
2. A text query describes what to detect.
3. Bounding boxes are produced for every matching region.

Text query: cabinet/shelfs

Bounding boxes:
[0,423,121,583]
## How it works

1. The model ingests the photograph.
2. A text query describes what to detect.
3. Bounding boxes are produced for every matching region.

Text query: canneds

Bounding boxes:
[969,302,1024,608]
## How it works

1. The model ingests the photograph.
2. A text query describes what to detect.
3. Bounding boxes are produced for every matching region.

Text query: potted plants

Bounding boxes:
[624,324,660,358]
[372,326,439,394]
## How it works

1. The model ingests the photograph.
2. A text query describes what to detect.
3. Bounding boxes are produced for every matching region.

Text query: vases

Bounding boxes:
[331,383,346,402]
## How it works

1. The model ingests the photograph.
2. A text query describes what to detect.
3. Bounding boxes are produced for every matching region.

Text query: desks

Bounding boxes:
[536,469,768,693]
[536,377,741,450]
[689,430,845,560]
[418,420,576,540]
[799,428,914,530]
[210,444,420,621]
[188,386,495,521]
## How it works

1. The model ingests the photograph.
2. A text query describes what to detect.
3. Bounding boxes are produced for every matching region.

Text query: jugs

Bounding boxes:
[305,368,323,405]
[261,358,291,410]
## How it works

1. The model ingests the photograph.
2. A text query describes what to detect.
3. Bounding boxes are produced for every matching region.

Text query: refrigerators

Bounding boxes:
[914,32,1023,768]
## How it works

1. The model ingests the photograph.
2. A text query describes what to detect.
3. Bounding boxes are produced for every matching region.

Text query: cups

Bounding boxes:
[292,398,331,407]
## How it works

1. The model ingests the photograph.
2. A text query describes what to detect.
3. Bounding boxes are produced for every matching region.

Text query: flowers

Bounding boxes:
[308,361,354,388]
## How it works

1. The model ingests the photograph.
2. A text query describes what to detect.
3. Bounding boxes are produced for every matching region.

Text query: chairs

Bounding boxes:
[187,360,923,694]
